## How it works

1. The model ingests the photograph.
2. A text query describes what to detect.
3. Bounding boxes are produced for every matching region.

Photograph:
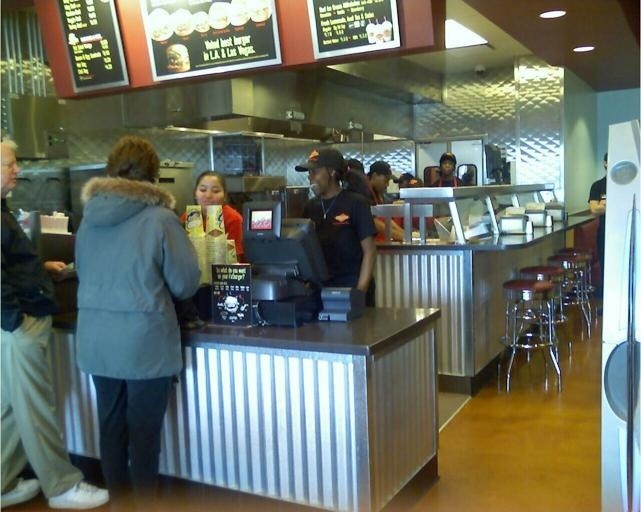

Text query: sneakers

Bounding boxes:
[2,478,41,510]
[47,481,111,510]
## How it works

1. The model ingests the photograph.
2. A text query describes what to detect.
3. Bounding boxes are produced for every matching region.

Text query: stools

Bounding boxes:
[500,245,596,397]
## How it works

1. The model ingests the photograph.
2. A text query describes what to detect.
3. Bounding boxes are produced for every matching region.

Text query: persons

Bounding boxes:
[1,132,110,512]
[179,171,245,263]
[295,147,377,308]
[75,135,203,512]
[341,152,464,243]
[588,153,608,316]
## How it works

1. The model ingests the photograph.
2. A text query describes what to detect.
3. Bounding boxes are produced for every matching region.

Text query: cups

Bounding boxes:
[186,204,228,285]
[227,240,238,267]
[40,215,69,234]
[365,22,394,44]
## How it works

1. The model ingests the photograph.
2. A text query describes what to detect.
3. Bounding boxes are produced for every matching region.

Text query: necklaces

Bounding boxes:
[320,194,339,219]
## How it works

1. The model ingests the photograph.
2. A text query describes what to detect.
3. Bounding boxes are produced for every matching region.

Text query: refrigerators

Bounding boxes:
[592,119,640,511]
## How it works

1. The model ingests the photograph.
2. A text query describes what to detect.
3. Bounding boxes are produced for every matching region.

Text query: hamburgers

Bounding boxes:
[166,43,191,73]
[149,0,271,42]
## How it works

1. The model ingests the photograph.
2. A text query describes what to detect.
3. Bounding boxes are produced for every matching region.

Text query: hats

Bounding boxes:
[438,151,457,166]
[294,147,350,174]
[392,172,415,184]
[366,159,394,177]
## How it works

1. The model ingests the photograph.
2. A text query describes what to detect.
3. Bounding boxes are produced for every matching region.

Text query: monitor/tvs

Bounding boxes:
[244,218,327,300]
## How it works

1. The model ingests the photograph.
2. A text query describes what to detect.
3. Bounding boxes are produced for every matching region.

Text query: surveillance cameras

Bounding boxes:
[474,65,486,75]
